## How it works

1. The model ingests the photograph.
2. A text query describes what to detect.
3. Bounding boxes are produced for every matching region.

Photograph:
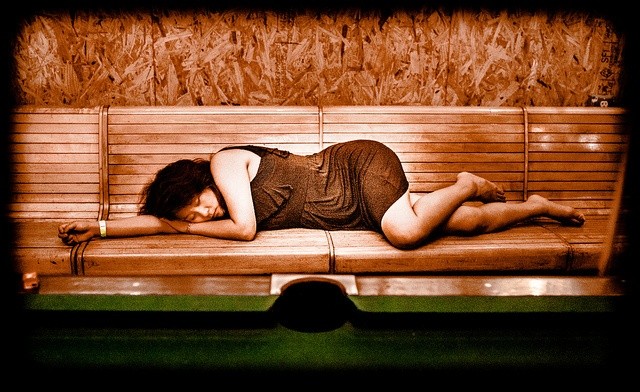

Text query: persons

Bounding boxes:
[58,140,586,251]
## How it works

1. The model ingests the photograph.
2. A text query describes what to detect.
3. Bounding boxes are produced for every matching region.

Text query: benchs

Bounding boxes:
[12,104,628,275]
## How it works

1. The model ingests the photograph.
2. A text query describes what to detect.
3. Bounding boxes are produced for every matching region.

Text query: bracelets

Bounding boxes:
[99,221,106,238]
